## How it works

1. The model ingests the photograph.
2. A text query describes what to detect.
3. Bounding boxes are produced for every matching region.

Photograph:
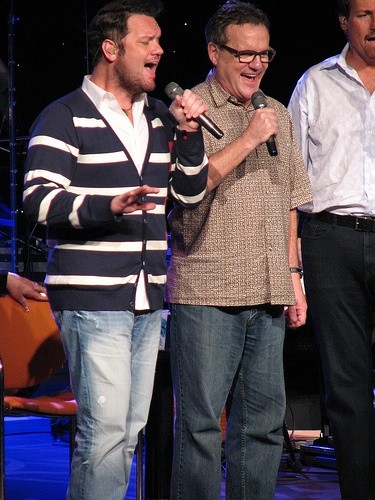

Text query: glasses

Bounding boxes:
[220,44,276,63]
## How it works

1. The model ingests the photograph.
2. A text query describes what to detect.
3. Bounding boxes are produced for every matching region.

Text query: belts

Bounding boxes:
[318,211,375,232]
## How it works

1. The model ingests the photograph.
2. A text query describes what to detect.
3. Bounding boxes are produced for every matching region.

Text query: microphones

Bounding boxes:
[164,82,224,140]
[251,91,278,157]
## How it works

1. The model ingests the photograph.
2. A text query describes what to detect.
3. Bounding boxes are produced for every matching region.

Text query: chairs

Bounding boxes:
[0,290,143,500]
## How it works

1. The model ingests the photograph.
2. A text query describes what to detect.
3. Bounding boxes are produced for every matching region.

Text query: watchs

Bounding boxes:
[289,267,304,279]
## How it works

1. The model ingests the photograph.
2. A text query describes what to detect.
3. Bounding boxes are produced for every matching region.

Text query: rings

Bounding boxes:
[200,100,204,104]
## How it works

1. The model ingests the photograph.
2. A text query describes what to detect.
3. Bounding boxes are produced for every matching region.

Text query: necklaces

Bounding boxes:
[122,102,133,116]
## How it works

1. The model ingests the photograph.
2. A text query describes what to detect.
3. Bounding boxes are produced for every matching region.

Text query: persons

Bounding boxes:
[287,0,375,500]
[174,306,227,500]
[167,0,315,500]
[23,0,209,500]
[0,270,49,312]
